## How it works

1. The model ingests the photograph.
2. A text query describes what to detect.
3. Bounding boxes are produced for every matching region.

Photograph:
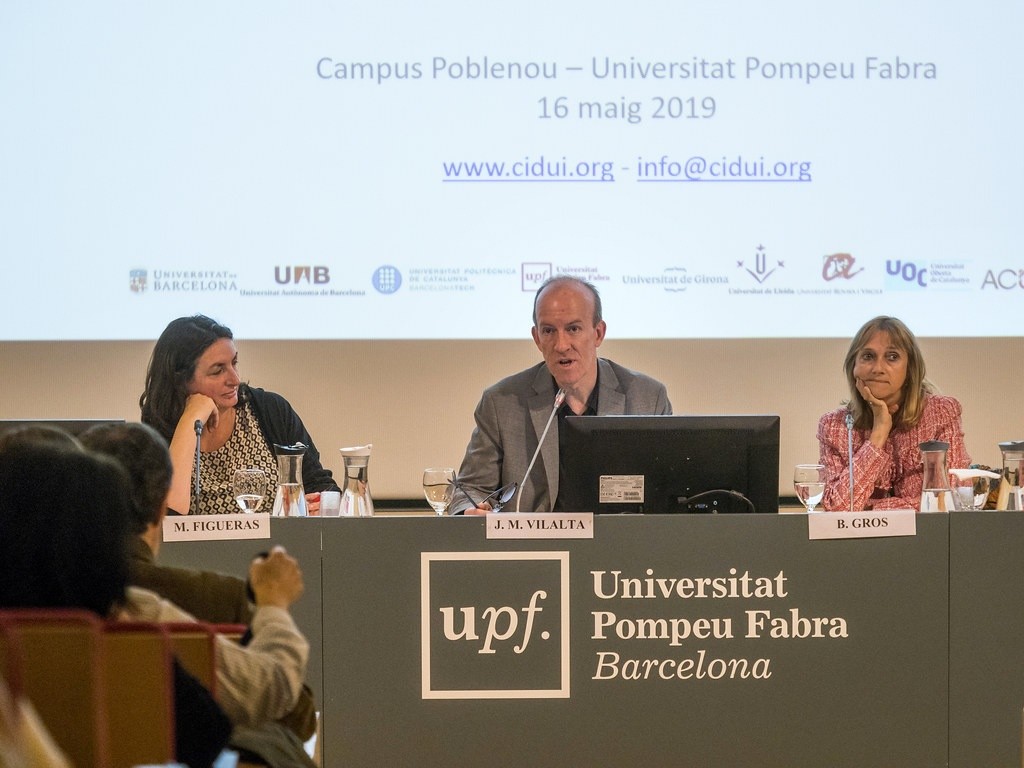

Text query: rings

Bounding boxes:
[870,402,872,404]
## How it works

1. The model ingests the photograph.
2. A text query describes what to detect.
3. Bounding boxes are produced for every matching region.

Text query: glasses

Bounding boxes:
[447,479,518,513]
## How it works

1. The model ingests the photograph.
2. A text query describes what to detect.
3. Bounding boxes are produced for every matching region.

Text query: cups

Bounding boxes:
[320,491,340,516]
[423,469,457,515]
[793,464,826,513]
[951,473,990,511]
[233,469,265,514]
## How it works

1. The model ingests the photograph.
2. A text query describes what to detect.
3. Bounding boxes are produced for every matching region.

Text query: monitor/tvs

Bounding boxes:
[0,420,126,443]
[563,414,781,514]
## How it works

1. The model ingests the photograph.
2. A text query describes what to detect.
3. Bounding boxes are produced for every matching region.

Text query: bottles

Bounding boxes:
[273,442,309,517]
[917,440,955,512]
[996,440,1024,510]
[338,444,374,516]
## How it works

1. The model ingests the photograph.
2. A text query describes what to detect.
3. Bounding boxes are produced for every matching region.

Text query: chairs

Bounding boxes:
[0,605,242,768]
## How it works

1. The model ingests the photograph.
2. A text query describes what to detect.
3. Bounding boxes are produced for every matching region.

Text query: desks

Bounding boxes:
[153,505,1024,768]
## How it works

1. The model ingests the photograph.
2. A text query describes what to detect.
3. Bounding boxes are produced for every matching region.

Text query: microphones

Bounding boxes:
[846,414,854,512]
[516,387,568,512]
[194,419,202,515]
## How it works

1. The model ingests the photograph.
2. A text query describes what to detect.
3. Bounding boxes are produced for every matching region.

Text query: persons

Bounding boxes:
[140,316,341,515]
[448,277,672,516]
[77,422,317,743]
[0,427,317,767]
[816,315,971,512]
